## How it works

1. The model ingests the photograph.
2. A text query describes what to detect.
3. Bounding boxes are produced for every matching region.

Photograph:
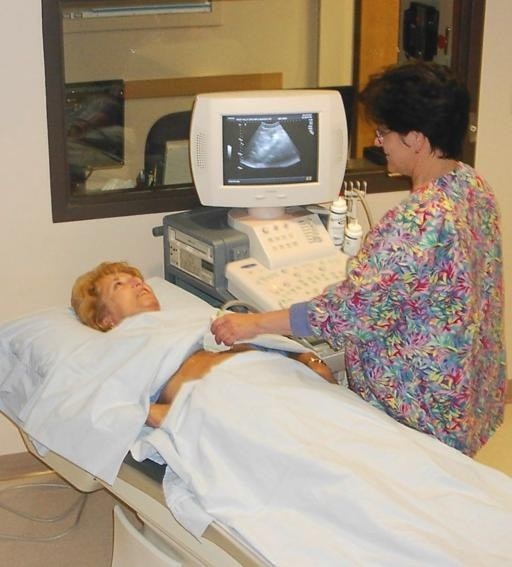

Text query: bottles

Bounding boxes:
[328,196,363,256]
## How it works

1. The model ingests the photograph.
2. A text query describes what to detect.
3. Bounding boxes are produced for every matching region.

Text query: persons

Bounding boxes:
[68,258,511,566]
[208,57,510,464]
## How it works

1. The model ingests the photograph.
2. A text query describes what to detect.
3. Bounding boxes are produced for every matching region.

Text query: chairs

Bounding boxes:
[144,109,193,186]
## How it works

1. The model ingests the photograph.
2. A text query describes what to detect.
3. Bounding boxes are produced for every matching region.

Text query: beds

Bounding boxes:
[3,360,279,567]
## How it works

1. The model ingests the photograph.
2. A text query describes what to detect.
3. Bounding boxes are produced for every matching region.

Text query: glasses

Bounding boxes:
[375,128,391,144]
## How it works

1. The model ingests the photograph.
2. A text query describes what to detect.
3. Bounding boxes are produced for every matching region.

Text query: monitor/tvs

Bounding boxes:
[64,79,124,174]
[189,90,349,207]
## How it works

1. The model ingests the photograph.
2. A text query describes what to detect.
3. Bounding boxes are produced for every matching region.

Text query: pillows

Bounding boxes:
[4,275,215,418]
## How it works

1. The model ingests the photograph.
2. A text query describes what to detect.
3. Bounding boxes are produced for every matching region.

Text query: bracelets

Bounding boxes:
[300,356,329,367]
